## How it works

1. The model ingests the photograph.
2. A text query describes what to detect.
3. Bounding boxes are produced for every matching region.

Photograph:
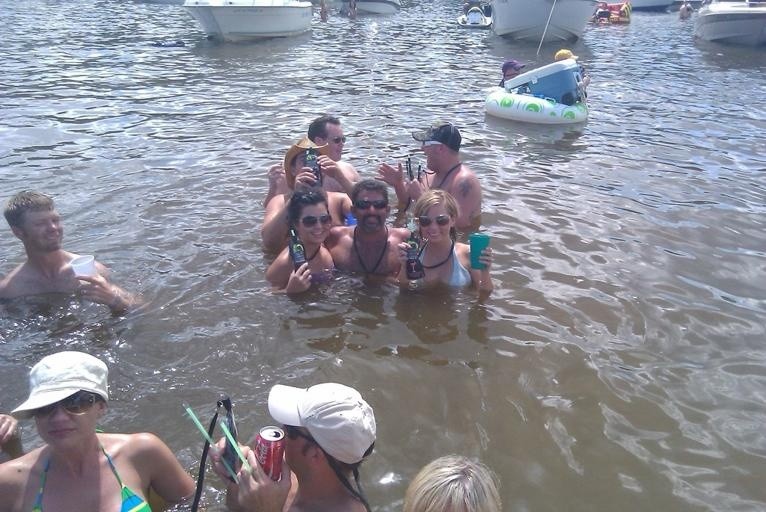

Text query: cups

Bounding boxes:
[69,254,96,286]
[468,233,491,270]
[303,149,321,184]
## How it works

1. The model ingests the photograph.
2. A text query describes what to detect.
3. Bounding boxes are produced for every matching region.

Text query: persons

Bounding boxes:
[403,456,503,512]
[0,414,26,457]
[500,60,524,88]
[680,1,692,19]
[318,0,338,21]
[1,189,129,314]
[556,47,590,97]
[347,2,358,20]
[208,382,376,511]
[0,351,198,511]
[262,117,496,295]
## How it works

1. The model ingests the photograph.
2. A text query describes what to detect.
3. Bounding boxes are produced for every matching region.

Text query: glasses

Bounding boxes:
[334,136,346,143]
[419,216,449,226]
[356,200,386,208]
[287,425,309,440]
[422,141,441,146]
[39,391,101,415]
[303,215,332,224]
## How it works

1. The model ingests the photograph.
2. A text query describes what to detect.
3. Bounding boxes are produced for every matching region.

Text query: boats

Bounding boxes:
[455,6,492,28]
[695,0,765,47]
[490,0,599,44]
[597,0,674,11]
[181,0,319,42]
[332,0,400,16]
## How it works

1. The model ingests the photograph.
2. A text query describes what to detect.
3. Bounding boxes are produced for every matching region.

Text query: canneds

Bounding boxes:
[252,426,285,481]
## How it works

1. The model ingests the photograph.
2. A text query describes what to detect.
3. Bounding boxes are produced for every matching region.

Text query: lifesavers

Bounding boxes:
[484,91,586,125]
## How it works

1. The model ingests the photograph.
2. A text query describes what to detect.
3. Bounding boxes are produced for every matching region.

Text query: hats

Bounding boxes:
[412,122,461,152]
[10,351,109,420]
[268,383,376,464]
[502,60,525,72]
[555,50,579,61]
[285,136,329,190]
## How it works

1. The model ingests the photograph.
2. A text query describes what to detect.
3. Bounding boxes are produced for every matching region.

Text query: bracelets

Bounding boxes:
[107,289,123,308]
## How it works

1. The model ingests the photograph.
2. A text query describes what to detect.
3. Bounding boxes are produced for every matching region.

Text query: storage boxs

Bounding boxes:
[503,57,583,104]
[468,9,481,24]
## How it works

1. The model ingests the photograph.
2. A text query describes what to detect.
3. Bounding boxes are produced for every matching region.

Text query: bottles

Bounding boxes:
[288,227,306,274]
[404,232,425,287]
